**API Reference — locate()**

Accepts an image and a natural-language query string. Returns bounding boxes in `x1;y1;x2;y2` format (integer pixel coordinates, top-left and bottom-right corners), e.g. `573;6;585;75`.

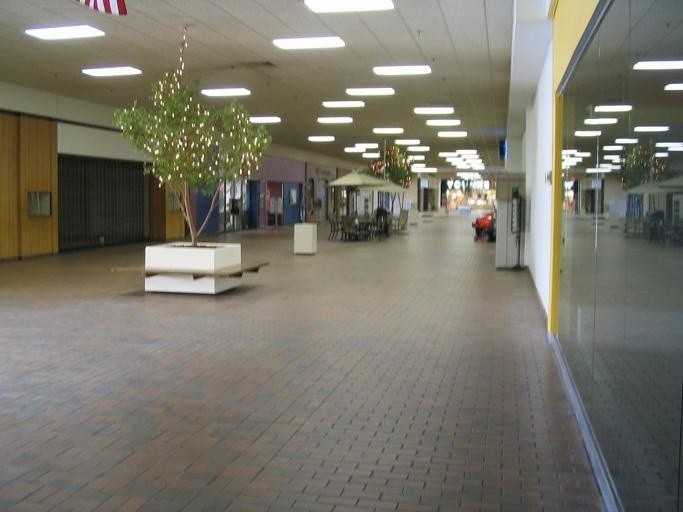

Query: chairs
327;210;410;241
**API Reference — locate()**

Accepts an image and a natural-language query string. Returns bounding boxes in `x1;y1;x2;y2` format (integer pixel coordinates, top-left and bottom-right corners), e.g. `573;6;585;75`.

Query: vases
146;239;242;296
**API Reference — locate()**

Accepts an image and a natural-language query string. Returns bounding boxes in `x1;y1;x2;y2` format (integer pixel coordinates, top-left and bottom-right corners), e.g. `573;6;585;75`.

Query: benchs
218;261;268;279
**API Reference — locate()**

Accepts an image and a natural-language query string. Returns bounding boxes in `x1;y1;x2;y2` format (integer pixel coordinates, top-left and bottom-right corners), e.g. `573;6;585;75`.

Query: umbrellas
658;175;683;189
328;171;383;217
354;181;409;209
626;180;683;223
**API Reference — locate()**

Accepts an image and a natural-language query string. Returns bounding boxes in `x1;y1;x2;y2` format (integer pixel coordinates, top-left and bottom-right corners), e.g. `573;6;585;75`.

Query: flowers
113;24;272;245
